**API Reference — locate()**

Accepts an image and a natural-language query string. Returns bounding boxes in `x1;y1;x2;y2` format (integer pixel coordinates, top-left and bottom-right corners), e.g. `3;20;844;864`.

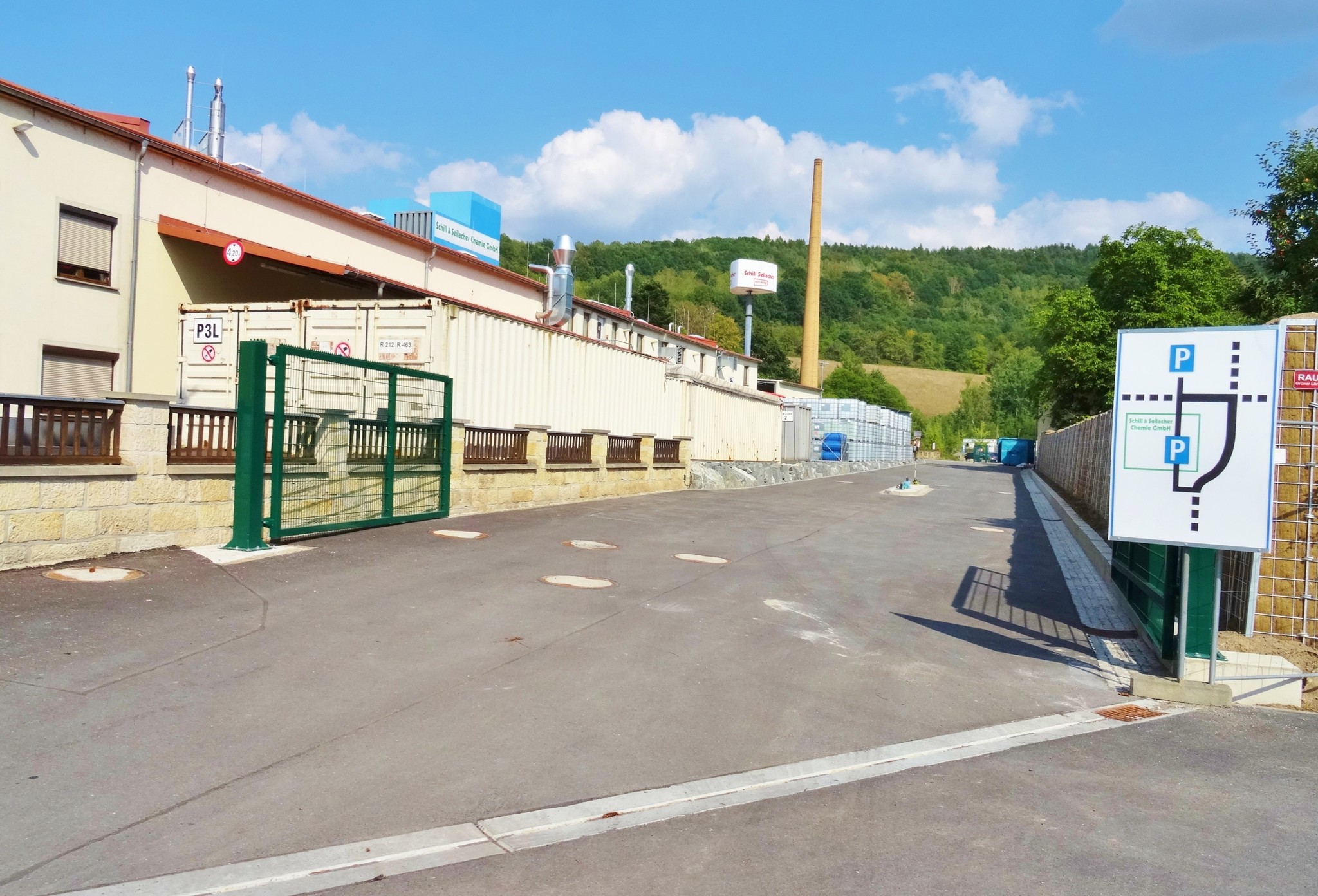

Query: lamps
12;120;34;133
320;277;362;290
258;258;309;278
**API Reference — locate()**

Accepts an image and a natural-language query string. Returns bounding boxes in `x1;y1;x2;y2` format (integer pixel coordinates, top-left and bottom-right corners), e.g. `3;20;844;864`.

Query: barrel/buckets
821;433;849;461
